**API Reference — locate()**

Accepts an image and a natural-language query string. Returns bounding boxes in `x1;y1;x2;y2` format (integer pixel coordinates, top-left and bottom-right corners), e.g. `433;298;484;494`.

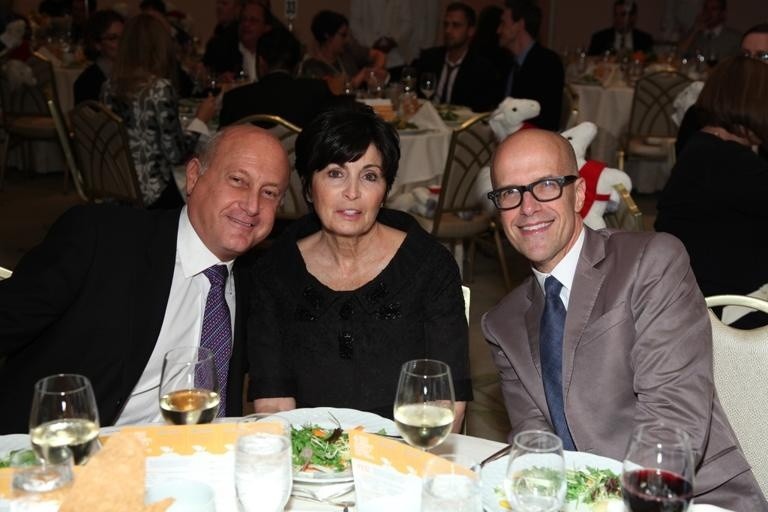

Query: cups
572;45;721;87
234;410;295;512
504;430;569;512
623;421;695;512
205;73;223;97
32;373;102;468
10;446;76;512
158;353;222;424
391;361;456;456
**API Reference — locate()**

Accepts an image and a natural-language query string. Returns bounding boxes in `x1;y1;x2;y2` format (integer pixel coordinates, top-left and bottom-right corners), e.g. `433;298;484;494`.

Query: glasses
744;51;768;61
488;175;577;211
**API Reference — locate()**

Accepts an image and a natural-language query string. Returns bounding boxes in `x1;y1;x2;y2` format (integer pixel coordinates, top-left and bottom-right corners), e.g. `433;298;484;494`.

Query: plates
254;404;408;485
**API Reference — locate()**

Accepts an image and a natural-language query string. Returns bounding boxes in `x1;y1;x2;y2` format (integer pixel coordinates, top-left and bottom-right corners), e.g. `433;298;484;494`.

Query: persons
648;53;768;330
0;124;290;436
479;127;767;510
382;1;564;130
585;1;766;59
246;93;476;435
1;2;386;210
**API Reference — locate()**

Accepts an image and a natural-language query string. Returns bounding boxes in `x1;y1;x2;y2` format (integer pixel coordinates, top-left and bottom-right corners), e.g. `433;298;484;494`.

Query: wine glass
421;453;486;512
343;68;464;135
477;450;647;512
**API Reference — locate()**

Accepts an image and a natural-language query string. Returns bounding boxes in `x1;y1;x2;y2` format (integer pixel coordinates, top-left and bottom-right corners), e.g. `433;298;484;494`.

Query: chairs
705;293;768;503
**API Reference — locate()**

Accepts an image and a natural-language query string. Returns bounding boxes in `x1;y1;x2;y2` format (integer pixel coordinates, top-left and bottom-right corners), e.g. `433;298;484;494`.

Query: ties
195;264;234;419
440;64;457;103
541;276;577;451
